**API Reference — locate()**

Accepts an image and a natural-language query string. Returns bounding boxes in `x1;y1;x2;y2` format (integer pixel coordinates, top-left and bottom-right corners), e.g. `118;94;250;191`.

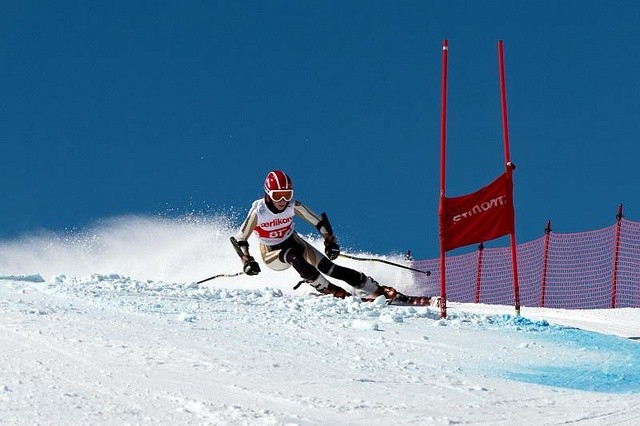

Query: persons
230;170;396;299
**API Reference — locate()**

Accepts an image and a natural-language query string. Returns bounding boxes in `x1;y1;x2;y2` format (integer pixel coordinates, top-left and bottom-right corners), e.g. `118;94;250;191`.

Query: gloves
325;243;340;260
243;259;261;275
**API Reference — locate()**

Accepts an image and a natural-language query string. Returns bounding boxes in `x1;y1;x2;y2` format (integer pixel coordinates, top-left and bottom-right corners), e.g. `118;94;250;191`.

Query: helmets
265;171;293;190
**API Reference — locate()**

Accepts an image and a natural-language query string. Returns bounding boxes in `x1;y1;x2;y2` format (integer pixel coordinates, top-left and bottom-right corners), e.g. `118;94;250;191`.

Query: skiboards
312;293;442;308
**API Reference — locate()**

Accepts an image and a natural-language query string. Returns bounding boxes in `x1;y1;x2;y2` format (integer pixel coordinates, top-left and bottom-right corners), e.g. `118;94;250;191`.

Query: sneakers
353;273;397;300
294;273;347;298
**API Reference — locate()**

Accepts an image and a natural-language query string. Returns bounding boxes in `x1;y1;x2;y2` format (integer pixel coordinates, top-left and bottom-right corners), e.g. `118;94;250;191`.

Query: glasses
265;190;294;202
